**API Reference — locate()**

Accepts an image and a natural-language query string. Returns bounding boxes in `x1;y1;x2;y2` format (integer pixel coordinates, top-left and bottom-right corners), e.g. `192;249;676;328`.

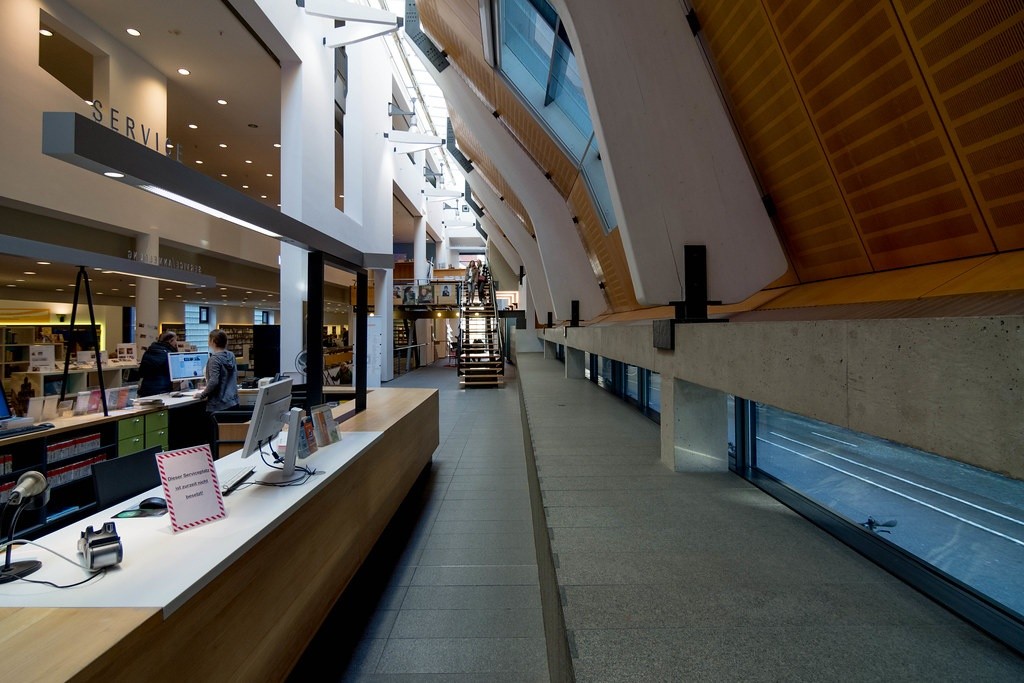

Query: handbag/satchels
478;275;486;281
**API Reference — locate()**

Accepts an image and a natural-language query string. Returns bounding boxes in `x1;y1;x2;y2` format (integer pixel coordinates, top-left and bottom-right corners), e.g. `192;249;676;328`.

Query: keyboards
218;465;256;494
0;425;49;439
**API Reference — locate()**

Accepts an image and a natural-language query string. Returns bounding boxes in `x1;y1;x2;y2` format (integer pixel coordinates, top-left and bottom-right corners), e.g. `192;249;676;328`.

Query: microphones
8;470;46;505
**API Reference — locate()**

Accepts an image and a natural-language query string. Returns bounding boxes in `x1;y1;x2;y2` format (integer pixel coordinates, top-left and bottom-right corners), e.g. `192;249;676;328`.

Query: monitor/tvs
0;379;12;420
167;351;209;391
240;377;308;486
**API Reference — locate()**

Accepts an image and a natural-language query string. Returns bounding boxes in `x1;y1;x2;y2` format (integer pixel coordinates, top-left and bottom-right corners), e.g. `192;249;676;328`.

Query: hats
159;331;176;342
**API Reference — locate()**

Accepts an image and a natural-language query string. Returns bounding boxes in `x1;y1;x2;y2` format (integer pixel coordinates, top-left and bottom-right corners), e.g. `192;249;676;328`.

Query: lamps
0;471;52;584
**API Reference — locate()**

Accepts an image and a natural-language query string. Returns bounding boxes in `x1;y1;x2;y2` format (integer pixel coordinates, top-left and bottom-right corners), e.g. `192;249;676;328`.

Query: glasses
409;291;413;294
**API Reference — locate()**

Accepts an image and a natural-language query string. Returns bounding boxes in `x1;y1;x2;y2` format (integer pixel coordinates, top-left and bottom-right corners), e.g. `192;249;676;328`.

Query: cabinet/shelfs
0;325;64;392
393;319;411;344
219;325;253;356
11;365;141;397
0;409;168;504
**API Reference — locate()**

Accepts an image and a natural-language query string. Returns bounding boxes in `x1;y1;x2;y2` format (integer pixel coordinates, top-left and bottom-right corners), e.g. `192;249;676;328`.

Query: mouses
40;422;55;428
139;497;167;508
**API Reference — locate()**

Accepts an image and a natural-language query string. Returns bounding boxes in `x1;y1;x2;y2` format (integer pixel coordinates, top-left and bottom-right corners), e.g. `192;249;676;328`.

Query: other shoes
471;304;475;307
466;300;470;305
479;303;484;307
484;298;487;304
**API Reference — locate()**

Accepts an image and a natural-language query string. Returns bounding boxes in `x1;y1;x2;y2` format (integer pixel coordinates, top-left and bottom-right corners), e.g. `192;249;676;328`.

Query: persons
193;329;241;420
135;330;179;398
341;328;348;347
476;260;487;306
330;361;352;385
402;287;414;304
442;286;449;296
465;260;478;306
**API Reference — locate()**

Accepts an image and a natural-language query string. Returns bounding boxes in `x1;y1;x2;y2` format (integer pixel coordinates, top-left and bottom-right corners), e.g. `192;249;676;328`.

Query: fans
295;349;326;376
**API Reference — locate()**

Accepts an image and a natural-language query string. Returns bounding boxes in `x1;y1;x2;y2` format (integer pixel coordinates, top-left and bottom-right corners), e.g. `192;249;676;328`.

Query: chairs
91;444;164;513
